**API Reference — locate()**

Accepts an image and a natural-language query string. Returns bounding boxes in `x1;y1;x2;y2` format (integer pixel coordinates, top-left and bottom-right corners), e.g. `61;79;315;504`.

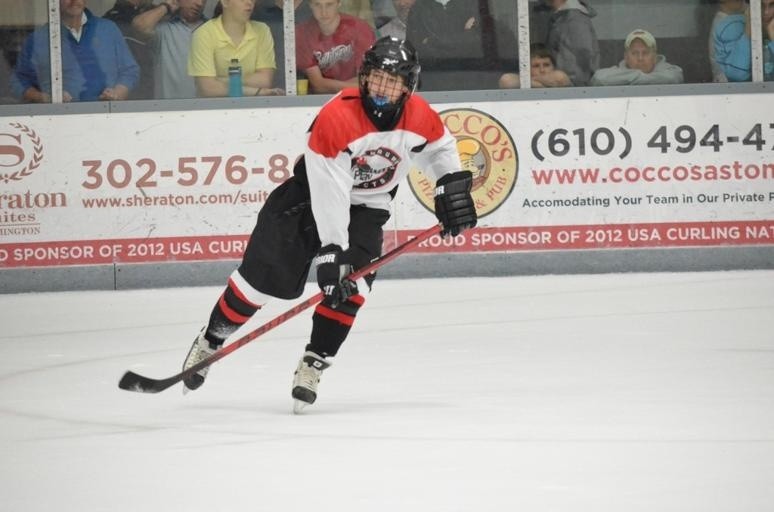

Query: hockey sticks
119;224;444;394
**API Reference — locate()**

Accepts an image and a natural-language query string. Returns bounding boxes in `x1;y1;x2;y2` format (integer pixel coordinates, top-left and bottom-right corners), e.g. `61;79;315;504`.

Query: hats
624;29;656;48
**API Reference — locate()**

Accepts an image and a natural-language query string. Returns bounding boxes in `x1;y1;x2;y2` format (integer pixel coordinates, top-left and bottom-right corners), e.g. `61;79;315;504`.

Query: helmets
359;35;419;122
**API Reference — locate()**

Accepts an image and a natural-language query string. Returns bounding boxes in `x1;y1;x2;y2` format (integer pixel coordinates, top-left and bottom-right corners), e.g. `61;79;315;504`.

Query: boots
182;328;224;390
291;344;330;404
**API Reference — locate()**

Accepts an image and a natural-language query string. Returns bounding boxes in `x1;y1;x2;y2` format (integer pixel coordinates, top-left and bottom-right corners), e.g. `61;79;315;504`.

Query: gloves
434;170;476;237
315;244;358;304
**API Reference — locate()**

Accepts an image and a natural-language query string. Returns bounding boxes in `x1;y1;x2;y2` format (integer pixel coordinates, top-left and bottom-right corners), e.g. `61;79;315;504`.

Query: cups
297;79;308;96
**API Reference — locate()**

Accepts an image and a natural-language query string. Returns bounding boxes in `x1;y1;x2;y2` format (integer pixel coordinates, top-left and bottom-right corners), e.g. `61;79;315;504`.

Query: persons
590;30;684;89
498;42;572;91
102;1;503;99
709;2;773;82
546;1;601;88
183;36;480;406
7;0;141;103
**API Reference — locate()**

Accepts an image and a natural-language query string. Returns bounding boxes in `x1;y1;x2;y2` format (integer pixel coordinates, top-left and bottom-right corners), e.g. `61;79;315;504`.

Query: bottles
228;59;243;97
